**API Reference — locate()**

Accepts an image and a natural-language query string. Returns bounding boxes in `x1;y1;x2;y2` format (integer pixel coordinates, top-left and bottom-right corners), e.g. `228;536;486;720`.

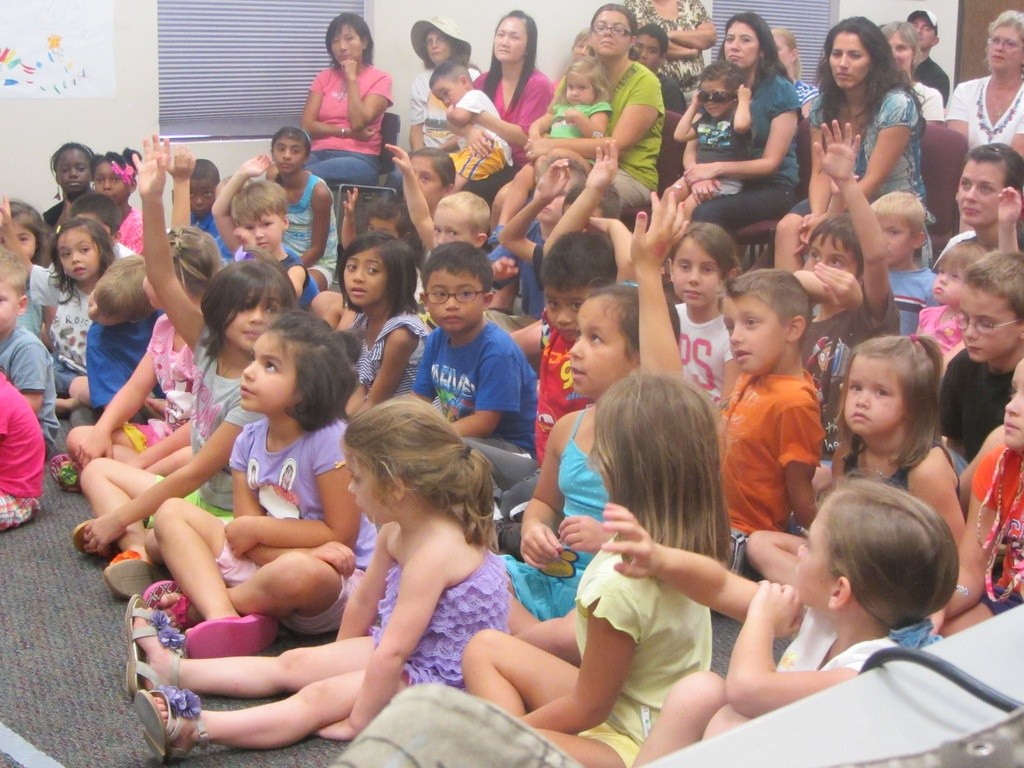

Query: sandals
134;686;209;763
125;593;185;697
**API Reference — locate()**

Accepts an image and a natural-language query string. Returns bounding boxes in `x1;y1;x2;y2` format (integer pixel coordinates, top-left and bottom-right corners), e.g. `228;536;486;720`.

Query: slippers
182;614;279;659
144;581;205;629
50;454;82;492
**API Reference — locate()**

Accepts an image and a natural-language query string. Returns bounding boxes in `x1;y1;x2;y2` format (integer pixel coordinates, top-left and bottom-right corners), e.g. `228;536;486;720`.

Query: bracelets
340;128;345;138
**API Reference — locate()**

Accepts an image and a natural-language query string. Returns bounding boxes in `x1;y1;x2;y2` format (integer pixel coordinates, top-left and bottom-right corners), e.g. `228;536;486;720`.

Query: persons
461;378;731;768
122;393;508;766
0;0;1024;766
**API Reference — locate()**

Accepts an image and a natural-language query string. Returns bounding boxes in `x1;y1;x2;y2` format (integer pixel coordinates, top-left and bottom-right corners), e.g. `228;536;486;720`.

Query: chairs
620;109;969;268
331;113;400;231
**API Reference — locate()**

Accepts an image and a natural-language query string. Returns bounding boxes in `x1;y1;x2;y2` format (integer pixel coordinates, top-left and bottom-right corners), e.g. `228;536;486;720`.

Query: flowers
112;162;134;186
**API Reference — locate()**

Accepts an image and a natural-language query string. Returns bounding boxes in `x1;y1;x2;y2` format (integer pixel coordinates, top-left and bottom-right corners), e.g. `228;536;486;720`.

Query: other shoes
104;551;167;600
70;408;96;426
72;519;121;558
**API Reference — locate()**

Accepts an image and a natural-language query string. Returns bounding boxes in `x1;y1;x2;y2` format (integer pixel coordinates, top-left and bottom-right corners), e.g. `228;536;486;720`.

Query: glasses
987;36;1022;49
592;25;633;36
698;91;736;102
954;312;1021;334
424;290;484;303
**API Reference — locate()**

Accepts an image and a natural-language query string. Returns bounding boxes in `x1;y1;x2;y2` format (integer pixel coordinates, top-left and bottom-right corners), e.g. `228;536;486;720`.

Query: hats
908;11;937;36
411;21;471;65
